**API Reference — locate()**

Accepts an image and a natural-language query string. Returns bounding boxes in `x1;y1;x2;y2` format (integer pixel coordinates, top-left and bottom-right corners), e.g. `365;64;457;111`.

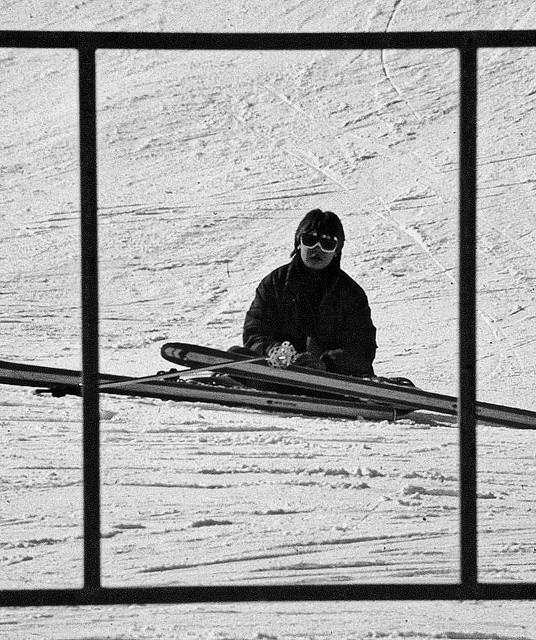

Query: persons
224;210;378;382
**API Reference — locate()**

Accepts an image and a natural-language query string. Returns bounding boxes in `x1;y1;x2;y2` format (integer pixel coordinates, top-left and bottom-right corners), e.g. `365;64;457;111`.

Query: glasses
298;231;339;254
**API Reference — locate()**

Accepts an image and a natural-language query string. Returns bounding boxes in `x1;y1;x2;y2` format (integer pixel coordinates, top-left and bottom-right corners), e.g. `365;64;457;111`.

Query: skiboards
0;342;534;431
